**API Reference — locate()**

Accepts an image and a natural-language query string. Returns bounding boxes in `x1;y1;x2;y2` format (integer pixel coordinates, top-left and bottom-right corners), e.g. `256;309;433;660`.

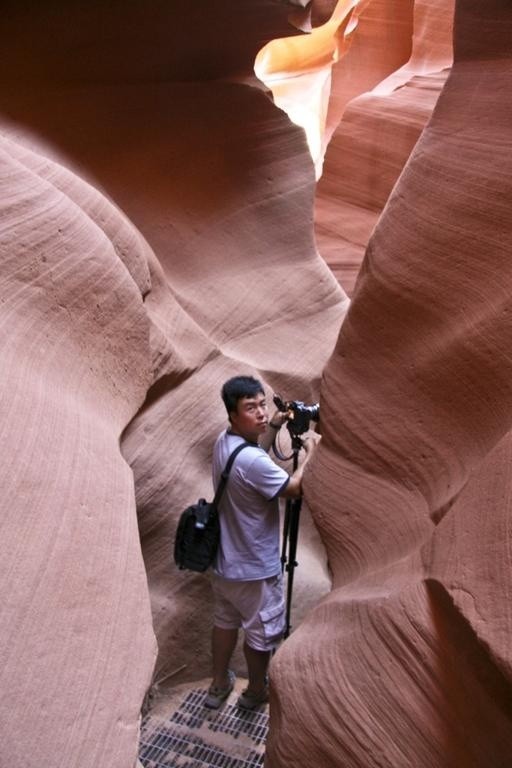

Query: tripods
280;438;304;640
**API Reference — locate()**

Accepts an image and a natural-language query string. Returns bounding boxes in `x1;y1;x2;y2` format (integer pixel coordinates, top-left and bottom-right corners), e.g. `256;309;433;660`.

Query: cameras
284;399;320;436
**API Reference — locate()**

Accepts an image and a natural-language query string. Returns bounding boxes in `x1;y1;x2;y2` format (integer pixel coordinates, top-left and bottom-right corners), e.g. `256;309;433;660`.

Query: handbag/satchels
173;441;256;574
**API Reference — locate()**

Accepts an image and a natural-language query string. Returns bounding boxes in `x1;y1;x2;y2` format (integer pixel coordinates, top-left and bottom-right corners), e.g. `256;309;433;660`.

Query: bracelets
270;421;283;432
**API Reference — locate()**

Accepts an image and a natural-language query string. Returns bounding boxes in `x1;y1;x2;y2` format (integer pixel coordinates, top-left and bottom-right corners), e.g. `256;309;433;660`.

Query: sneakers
235;675;270;711
203;666;235;710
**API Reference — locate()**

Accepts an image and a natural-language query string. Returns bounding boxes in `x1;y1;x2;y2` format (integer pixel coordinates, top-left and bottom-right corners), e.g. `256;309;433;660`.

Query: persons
199;373;313;713
